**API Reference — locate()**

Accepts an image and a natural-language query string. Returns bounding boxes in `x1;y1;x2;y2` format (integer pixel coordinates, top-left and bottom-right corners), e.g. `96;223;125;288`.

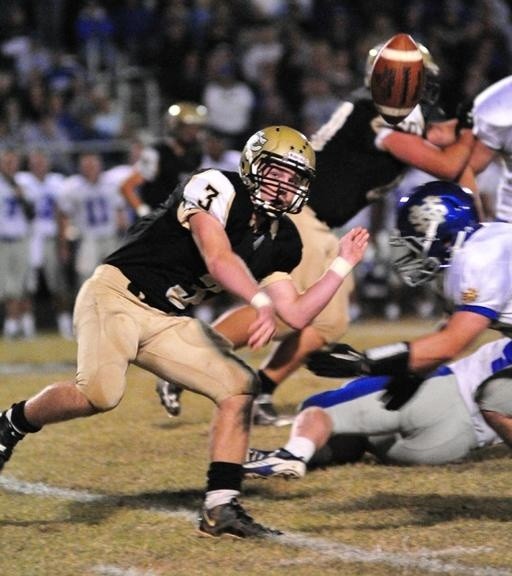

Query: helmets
163;101;209;144
238;125;317;217
387;180;479;287
363;39;441;110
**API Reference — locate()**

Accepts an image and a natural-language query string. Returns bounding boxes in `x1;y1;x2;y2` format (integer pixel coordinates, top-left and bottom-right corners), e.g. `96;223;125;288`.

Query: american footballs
370;34;425;125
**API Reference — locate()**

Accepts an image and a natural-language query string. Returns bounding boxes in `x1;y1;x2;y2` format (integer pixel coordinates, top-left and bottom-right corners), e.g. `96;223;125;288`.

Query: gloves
380;373;423;412
301;341;410;378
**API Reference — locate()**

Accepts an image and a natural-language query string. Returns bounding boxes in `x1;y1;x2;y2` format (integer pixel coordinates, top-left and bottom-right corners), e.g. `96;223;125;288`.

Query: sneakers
250;394;295;428
156;379;183;415
197;497;282;541
242;447;308;479
0;408;24;470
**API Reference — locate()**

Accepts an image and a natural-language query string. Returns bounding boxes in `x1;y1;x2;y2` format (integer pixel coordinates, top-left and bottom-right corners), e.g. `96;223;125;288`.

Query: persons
155;36;512;477
1;125;369;539
0;0;512;338
